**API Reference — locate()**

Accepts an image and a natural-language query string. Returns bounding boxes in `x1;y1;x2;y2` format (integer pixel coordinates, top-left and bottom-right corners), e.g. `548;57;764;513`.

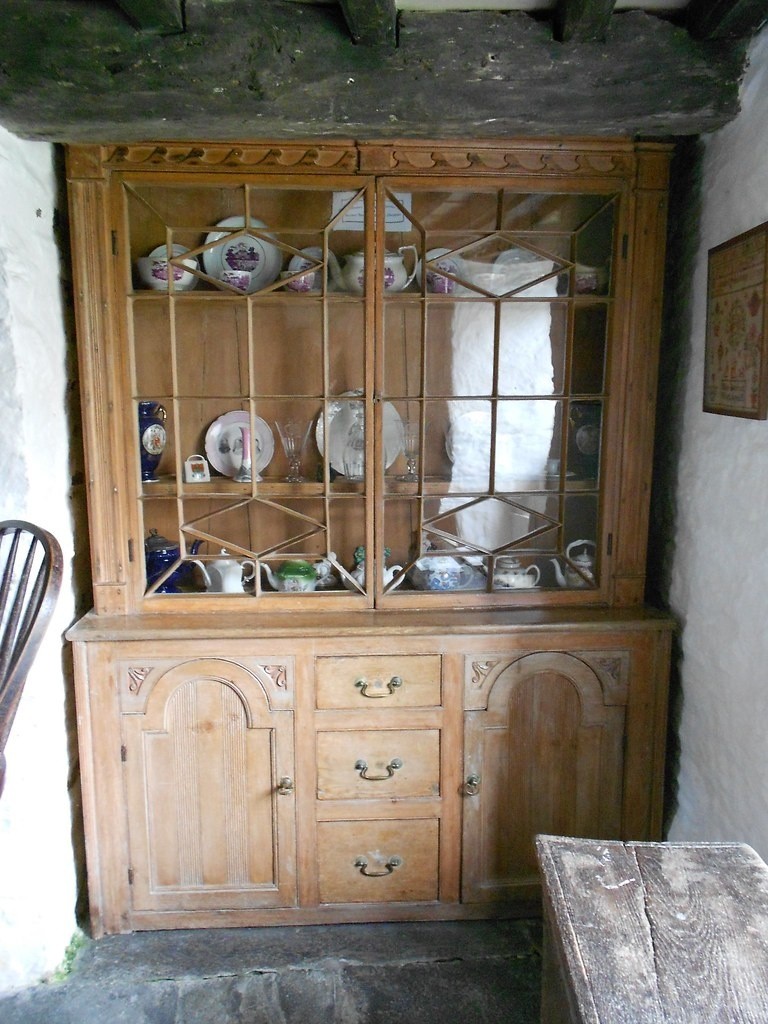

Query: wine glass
395;420;427;482
274;420;313;483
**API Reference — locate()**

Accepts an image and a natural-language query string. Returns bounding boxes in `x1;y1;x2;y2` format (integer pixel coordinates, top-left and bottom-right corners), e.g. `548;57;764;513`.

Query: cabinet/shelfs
60;137;679;922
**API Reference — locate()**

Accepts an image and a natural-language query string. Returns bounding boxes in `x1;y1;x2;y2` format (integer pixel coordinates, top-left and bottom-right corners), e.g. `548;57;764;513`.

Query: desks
533;834;768;1024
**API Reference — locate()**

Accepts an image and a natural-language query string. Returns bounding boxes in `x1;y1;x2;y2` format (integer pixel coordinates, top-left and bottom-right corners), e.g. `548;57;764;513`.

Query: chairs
0;519;62;798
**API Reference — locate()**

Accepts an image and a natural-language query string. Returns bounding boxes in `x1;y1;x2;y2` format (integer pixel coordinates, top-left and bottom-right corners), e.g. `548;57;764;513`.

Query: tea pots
318;242;417;292
144;528;599;593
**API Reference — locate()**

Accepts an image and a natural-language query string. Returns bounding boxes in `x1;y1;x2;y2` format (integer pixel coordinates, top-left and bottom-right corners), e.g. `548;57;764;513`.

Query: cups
547;459;560;475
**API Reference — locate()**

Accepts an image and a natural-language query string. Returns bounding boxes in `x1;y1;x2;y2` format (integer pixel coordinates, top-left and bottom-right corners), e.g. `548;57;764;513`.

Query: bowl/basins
431;273;456;293
138;258;197;290
218;271;251;292
280;271;315;292
575;267;603;295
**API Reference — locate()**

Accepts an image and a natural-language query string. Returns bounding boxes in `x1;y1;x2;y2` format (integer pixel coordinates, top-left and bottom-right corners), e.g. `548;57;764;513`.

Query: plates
446;412;491;477
492;248;538;296
149;244;200;290
416;248;468;293
289;247;338;292
544;471;575;478
316;391;403;475
203;216;282;294
205;410;274;476
